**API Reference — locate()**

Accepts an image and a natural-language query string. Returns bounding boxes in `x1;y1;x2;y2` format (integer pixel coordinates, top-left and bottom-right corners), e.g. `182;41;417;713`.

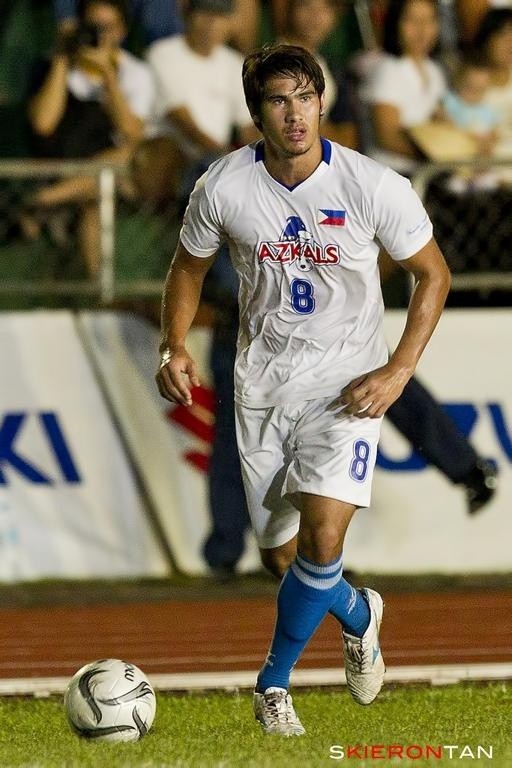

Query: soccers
63;657;157;745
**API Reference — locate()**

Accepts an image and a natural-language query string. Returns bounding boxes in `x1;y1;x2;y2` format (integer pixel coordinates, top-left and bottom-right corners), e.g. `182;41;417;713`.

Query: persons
2;0;512;281
153;36;451;739
175;117;502;580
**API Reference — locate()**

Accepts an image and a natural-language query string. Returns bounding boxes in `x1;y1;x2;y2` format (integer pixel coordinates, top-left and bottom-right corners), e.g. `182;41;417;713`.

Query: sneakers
250;684;307;738
337;584;388;707
